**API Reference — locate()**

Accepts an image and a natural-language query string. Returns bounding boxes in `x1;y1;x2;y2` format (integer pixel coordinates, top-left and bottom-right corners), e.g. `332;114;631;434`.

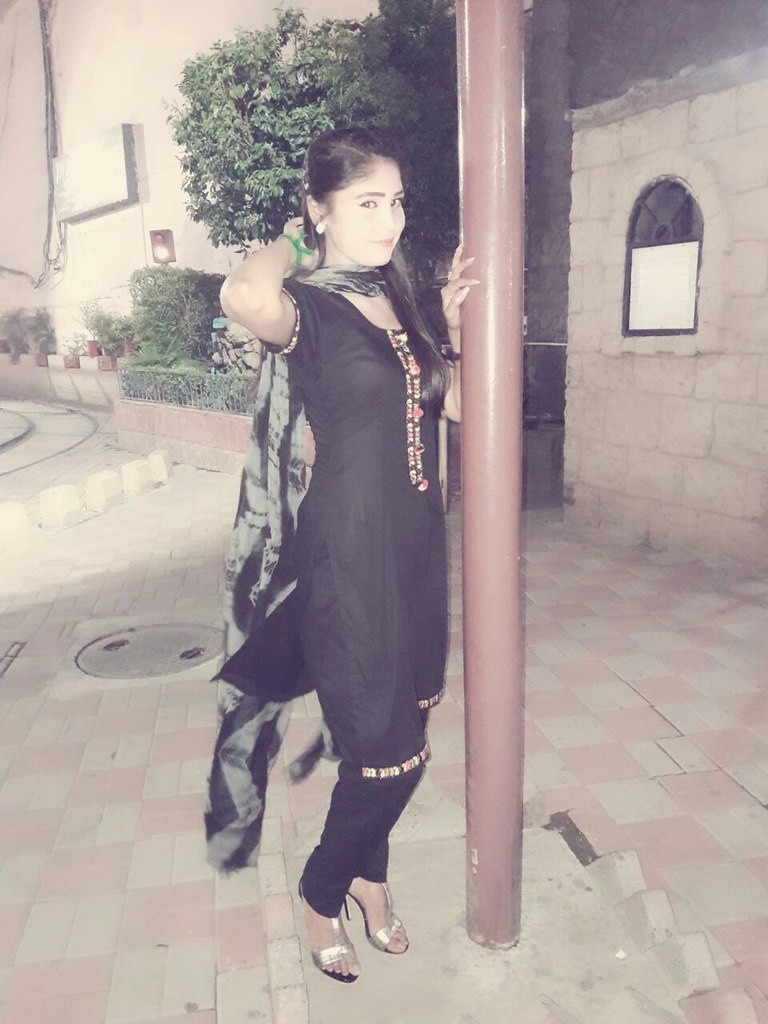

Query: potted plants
80;297;141;373
0;309;24;367
24;305;57;367
60;336;81;370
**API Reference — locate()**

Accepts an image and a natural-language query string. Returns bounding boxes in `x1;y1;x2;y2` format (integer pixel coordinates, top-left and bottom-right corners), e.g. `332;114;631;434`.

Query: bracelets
278;232;313;266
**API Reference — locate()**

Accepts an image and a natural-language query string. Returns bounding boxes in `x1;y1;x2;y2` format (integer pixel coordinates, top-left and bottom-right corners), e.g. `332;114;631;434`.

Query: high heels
299;876;358;984
344;883;409;954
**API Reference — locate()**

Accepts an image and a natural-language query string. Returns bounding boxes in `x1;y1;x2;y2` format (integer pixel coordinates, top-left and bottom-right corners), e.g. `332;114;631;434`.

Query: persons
204;126;480;986
